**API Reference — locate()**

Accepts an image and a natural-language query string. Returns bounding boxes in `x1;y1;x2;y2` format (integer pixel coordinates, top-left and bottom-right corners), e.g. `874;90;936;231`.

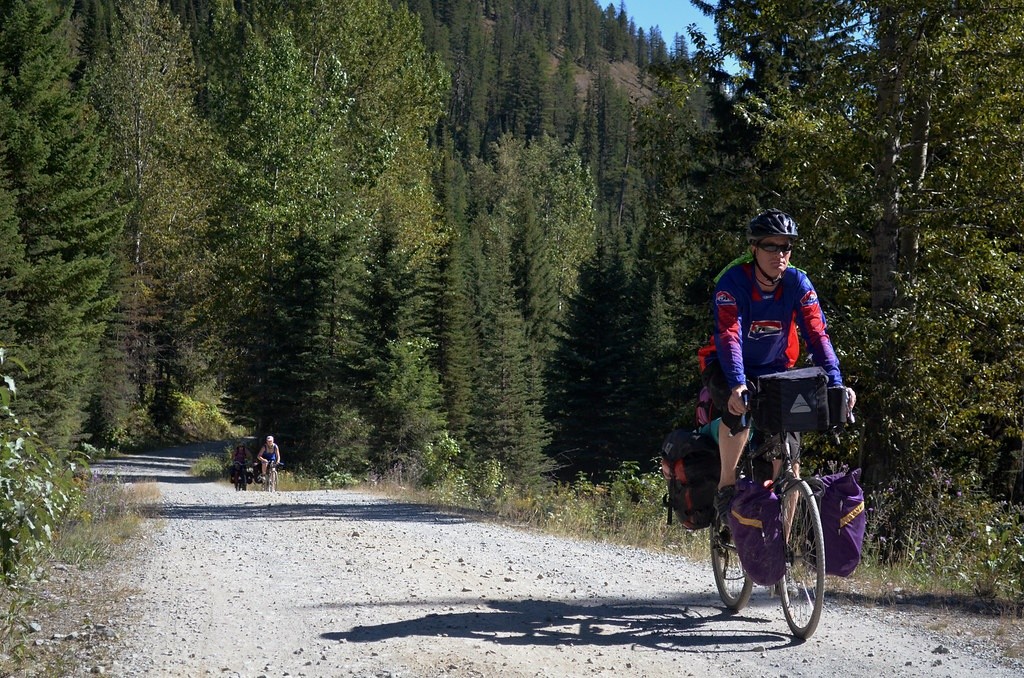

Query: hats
267;436;274;442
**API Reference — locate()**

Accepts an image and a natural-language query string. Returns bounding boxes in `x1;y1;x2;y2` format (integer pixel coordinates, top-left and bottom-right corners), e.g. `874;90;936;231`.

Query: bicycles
260;458;278;493
232;460;250;491
709;374;856;641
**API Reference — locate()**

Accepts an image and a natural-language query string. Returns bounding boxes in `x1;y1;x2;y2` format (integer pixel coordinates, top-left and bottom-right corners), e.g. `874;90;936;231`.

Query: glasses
750;242;793;252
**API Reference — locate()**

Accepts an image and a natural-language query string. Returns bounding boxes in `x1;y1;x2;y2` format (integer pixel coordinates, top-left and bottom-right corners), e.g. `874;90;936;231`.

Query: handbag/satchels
726;477;788;586
661;430;722;530
757;366;829;434
804;468;865;577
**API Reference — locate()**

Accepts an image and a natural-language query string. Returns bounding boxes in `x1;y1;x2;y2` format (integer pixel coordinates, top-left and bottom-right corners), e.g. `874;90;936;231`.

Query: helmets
746;208;798;246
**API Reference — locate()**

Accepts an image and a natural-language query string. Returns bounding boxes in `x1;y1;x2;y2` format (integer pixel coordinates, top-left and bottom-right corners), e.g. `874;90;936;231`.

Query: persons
232;441;253;482
702;206;859;573
257;435;281;485
